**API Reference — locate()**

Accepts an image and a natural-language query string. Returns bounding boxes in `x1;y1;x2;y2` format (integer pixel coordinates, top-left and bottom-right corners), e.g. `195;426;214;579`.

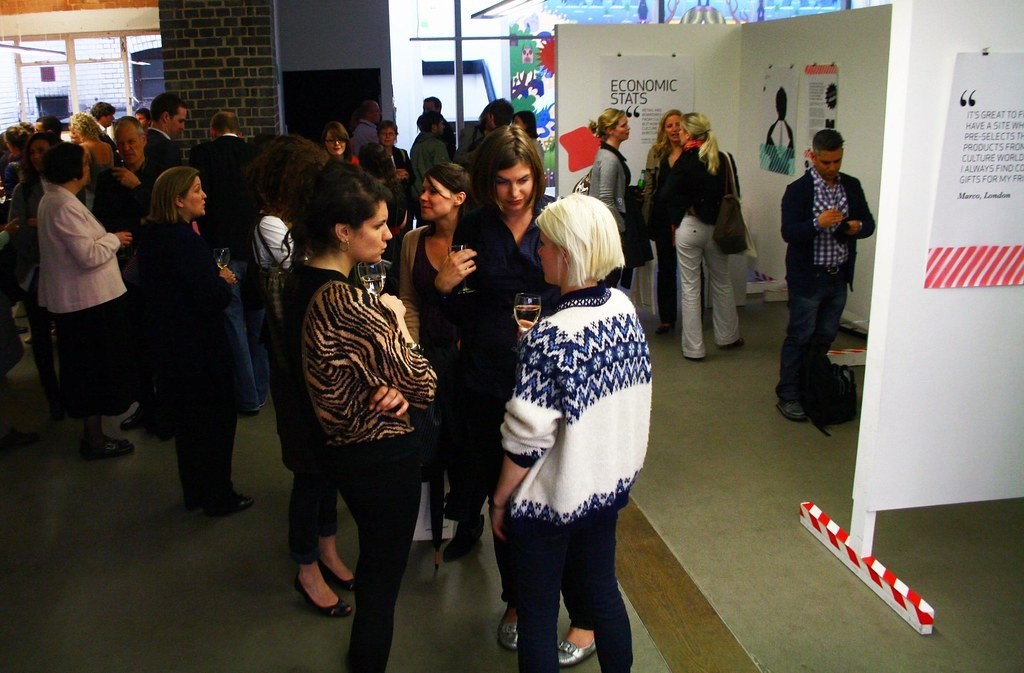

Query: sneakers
777;399;806;419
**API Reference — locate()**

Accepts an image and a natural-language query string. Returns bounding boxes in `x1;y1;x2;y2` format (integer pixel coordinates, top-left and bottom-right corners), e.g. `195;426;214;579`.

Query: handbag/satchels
712;150;749;254
240;260;275;297
801;354;856;436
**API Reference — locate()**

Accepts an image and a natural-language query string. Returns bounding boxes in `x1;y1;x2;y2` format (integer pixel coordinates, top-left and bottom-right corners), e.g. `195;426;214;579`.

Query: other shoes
0;427;40;447
204;494;253;516
497;607;519;650
655;323;672;334
442;514;485;561
15;327;27;334
557;637;596;666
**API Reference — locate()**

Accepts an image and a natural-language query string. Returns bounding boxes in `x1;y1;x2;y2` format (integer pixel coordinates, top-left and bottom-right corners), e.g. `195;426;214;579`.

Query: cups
357;259;386;296
213;248;230;269
111;159;124;167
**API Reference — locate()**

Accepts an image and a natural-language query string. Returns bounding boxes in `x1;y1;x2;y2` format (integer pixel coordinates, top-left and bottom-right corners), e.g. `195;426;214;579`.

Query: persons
492;193;652;673
0;92;187;460
643;109;705;334
587;109;632;288
270;158;437;673
135;166;259;517
431;125;596;666
776;129;876;420
657;113;745;360
189;110;331;280
399;162;485;561
324;97;543;236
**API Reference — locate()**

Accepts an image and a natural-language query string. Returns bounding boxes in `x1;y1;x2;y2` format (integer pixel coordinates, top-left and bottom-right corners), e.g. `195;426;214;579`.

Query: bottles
638;170;645;188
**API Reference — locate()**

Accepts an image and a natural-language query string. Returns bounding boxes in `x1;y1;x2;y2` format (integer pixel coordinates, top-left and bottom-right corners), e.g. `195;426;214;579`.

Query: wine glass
511;293;541;352
448;245;475;294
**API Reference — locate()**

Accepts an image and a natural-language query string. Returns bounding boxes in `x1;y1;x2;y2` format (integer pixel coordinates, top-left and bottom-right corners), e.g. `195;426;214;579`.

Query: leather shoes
121;407;147;428
79;436;134;459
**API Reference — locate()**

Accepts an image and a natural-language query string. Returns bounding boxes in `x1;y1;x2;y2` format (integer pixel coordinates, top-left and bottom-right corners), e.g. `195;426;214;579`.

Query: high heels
316;553;355;590
294;577;351;616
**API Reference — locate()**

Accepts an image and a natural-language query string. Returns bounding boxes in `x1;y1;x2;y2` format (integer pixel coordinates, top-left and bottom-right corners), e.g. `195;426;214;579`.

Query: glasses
325;138;344;143
